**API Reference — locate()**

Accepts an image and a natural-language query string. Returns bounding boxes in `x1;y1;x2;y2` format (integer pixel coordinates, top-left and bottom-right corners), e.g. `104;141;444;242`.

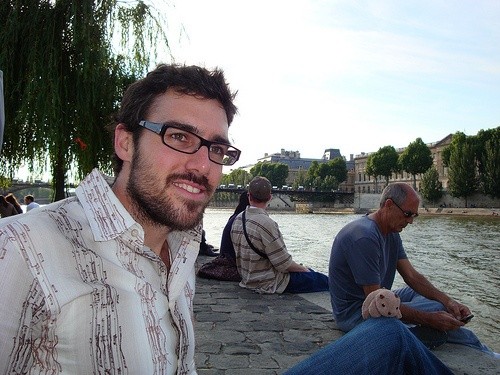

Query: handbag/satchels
198;253;241;281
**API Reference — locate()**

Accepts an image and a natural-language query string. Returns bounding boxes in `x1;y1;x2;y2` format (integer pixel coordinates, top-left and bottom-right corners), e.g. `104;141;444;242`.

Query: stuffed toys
362;289;402;320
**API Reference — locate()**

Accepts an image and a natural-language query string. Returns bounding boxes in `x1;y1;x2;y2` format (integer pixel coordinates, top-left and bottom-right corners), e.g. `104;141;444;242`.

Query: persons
231;176;328;294
328;182;500;358
5;193;23;214
220;192;250;259
0;195;17;218
0;65;456;375
24;195;39;211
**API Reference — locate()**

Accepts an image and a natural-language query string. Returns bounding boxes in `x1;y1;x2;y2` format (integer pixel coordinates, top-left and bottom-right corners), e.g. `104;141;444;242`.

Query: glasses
139;120;241;166
385;197;419;218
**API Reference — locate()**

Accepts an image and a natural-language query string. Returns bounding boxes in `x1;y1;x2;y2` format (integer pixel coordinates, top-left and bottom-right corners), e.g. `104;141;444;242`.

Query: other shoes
408;325;447;349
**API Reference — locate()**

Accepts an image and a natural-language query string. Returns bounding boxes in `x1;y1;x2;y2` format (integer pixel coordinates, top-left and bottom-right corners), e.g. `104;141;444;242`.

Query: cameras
459;314;474;322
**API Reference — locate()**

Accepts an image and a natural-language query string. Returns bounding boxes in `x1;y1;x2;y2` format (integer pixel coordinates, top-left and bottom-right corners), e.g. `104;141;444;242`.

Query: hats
249;176;271;199
